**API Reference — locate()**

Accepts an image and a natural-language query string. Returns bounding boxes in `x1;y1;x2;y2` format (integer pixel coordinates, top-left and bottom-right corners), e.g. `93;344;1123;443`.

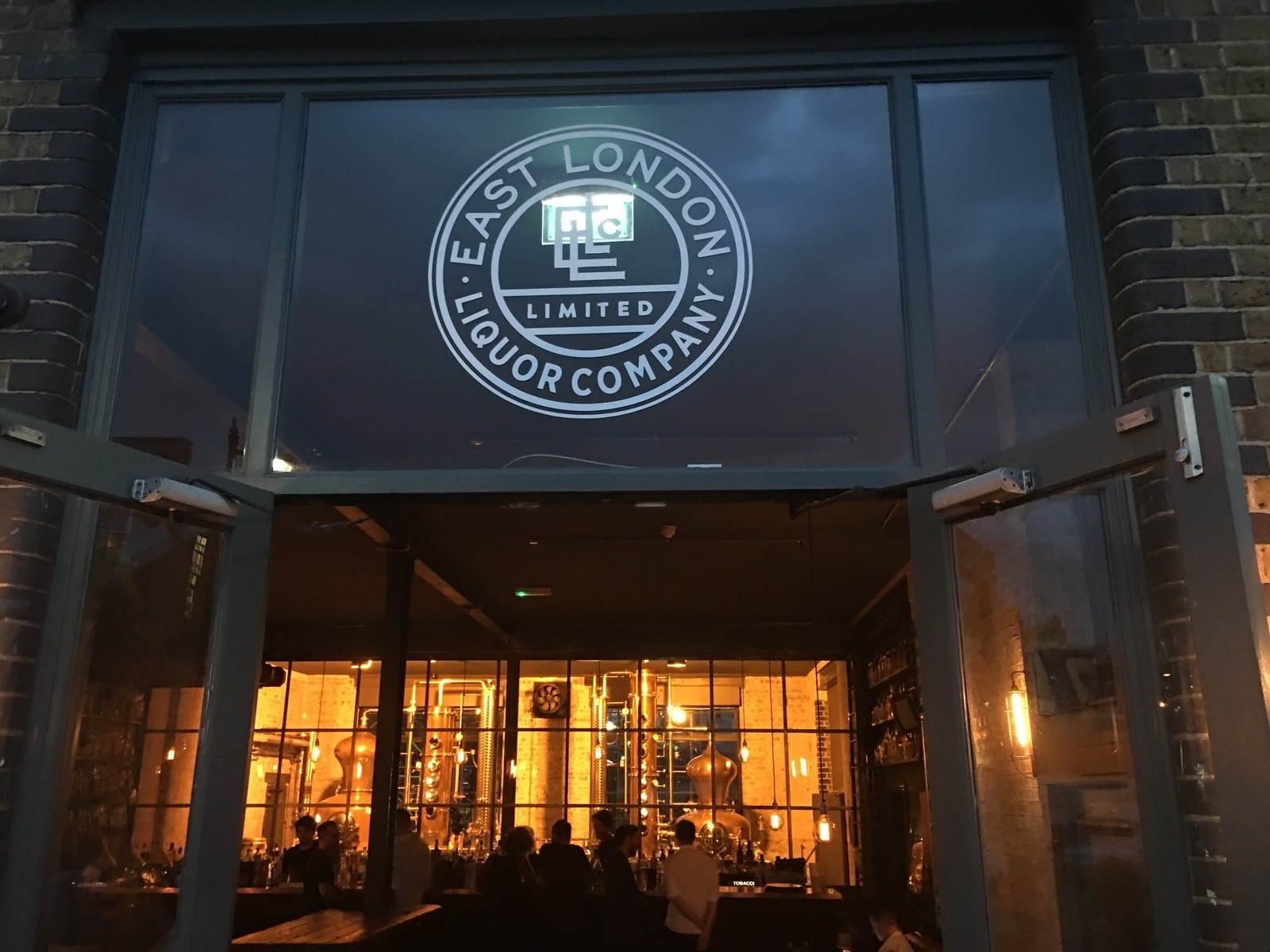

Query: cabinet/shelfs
867;663;922;768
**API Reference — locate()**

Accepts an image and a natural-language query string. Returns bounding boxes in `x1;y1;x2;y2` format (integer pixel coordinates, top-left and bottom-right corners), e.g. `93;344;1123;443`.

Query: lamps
1004;671;1038;780
738;661;751;764
812;661;837;844
767;660;784;830
454;660;468;765
310;662;322;762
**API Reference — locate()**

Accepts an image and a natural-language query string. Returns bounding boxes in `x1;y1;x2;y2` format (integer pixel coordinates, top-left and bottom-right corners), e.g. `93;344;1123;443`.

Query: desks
231;904;441;952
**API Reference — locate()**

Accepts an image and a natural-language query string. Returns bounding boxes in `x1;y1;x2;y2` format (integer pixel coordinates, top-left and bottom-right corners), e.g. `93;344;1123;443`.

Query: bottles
240;843;369;891
584;846;591;862
713;839;783;882
866;638;924;765
428;836;501;866
133;842;183;863
639;848;677;893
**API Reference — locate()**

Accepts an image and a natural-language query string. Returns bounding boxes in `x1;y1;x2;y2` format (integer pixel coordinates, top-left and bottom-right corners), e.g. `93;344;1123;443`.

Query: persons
392;808;434;906
475;827;550;952
660;819;720;952
838;899;915;952
268;816;319;886
539;819;589;952
589;809;652;952
303;821;340;914
